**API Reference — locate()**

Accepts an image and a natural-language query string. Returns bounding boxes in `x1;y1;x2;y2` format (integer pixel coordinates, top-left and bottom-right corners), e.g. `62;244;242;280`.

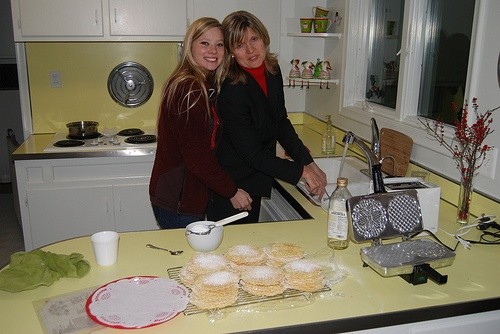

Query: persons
206;10;327;225
149;17;252;228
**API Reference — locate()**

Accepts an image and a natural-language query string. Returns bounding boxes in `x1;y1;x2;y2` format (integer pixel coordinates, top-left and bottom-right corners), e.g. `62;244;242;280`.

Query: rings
248;197;251;201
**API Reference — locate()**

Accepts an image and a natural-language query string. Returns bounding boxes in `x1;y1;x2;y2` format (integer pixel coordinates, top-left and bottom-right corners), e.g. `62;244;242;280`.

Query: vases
456;177;473;224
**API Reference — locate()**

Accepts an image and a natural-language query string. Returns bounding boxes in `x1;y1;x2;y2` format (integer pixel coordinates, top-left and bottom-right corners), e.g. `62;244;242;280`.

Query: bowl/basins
185;220;223;251
67;120;100;137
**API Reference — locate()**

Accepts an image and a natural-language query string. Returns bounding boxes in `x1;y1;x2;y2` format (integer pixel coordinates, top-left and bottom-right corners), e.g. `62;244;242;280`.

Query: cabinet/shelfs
11;0;349;86
15;160;305;254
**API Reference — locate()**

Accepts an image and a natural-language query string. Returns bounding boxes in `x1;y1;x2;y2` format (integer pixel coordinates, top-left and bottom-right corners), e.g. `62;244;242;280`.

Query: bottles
321;115;336;155
327;177;353;250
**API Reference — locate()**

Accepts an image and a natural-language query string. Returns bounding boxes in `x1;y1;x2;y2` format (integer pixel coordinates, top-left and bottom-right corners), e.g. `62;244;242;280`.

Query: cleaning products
320;114;336;155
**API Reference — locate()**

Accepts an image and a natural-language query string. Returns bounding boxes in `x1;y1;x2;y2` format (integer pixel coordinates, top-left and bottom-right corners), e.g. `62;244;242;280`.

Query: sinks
289;158;363;184
322;182;371;212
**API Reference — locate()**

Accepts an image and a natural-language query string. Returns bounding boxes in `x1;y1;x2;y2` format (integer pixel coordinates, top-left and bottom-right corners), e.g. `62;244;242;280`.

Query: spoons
147;244;183;255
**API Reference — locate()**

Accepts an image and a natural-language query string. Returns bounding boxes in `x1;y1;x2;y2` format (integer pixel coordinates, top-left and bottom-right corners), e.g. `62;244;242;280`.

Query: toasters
371;176;442;236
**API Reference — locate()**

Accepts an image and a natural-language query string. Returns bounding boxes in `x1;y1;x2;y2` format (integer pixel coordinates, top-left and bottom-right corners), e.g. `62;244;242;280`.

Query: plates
86;276;181;329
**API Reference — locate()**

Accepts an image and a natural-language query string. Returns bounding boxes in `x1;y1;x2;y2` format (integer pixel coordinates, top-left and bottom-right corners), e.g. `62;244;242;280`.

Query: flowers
417;97;500;220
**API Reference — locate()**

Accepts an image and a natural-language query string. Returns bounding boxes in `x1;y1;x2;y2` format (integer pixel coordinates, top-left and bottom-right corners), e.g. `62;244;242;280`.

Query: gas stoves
44;128;159;149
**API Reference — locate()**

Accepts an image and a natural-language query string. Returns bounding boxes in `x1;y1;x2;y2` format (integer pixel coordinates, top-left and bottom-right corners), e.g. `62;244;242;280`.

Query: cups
91;231;119;266
300;6;333;35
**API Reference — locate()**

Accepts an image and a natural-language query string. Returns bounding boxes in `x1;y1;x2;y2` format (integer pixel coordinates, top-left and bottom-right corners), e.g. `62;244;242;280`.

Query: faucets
342;117;381;175
